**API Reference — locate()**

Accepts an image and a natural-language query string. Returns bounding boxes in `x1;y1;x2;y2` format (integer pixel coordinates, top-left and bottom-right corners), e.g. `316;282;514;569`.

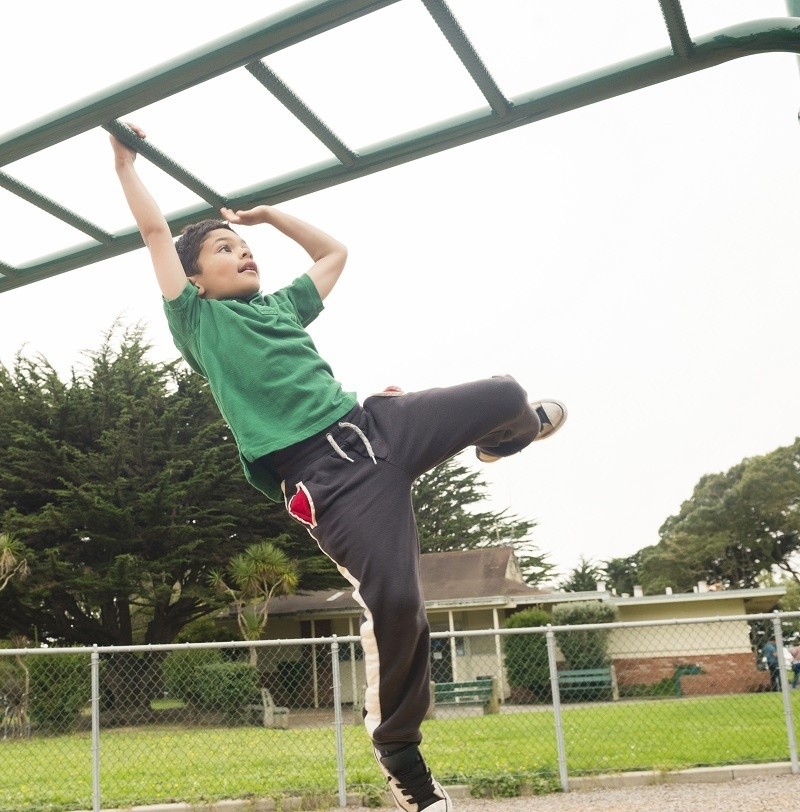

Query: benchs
557;665;620;701
424;677;499;718
244;688;290;729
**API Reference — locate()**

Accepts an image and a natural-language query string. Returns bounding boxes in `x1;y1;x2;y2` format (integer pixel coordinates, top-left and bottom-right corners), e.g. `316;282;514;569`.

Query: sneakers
475;402;566;463
373;742;452;812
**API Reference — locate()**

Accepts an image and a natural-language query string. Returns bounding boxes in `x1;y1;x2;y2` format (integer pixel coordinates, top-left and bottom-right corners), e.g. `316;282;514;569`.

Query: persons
764;636;782;692
108;122;567;812
779;640;794;672
790;638;800;689
